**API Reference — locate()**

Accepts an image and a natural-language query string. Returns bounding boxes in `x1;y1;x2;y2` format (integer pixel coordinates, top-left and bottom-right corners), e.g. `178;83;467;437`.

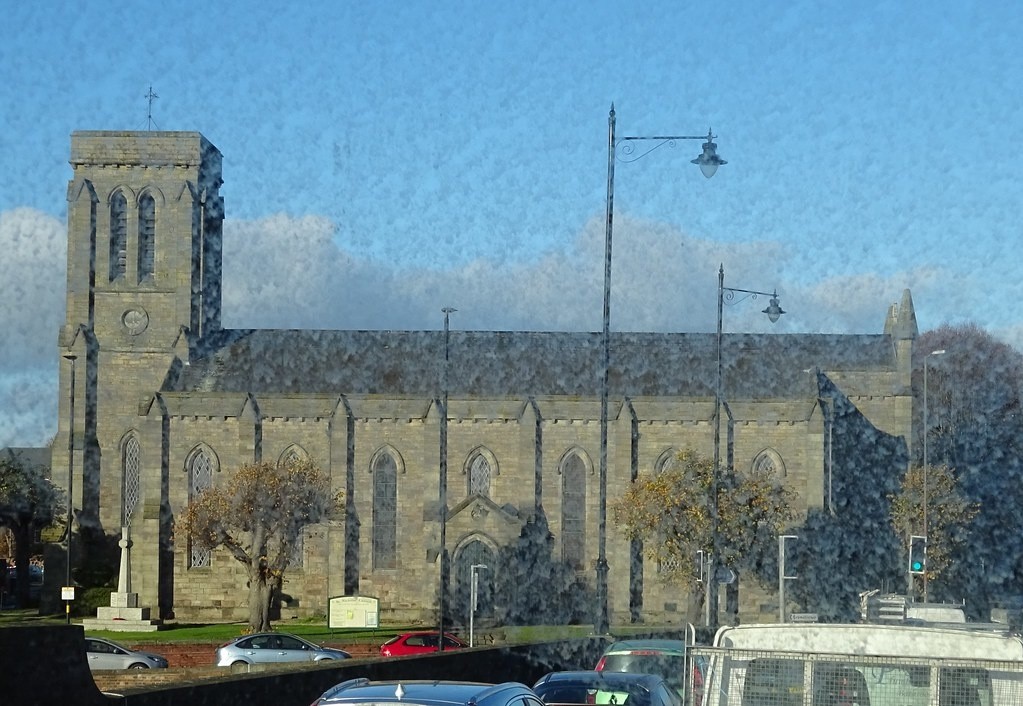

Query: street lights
920;342;947;607
63;346;76;585
440;306;453;650
595;101;728;636
713;262;783;623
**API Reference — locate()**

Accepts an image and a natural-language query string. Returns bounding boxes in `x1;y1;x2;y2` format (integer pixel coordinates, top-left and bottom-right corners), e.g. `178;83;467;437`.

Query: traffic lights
909;536;928;579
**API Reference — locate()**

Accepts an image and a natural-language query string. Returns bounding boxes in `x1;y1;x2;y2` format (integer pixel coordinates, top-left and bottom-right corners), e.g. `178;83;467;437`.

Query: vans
699;617;1016;706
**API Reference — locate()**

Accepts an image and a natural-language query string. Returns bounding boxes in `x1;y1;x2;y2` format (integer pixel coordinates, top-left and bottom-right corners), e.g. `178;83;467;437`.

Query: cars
209;635;350;670
534;671;686;705
594;639;709;697
87;636;167;675
378;630;469;655
313;679;541;706
